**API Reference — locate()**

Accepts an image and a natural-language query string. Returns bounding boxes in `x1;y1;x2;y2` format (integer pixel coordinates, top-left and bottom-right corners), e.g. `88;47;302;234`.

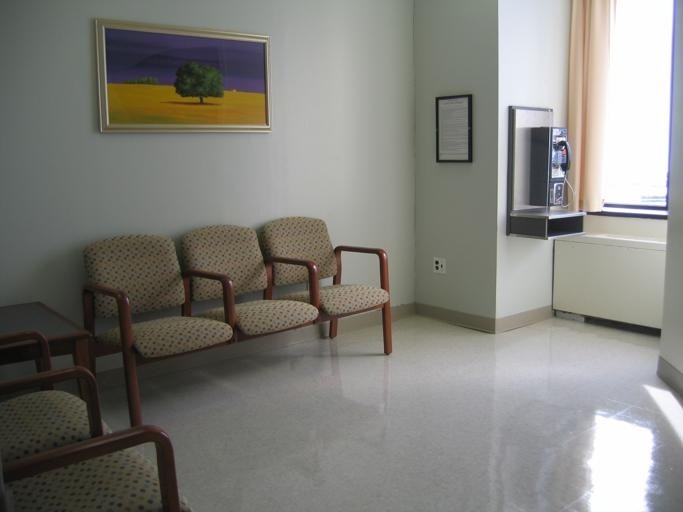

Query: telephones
550;127;571;182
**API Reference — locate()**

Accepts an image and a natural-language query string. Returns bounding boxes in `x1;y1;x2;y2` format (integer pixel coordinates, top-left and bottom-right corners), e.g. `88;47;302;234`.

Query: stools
0;329;190;512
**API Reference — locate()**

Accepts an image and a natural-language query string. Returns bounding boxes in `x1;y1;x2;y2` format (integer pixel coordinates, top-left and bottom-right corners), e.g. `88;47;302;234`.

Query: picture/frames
433;93;472;164
92;16;275;136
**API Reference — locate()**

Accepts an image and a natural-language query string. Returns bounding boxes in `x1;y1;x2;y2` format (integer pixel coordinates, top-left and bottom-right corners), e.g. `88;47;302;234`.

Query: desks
1;300;94;399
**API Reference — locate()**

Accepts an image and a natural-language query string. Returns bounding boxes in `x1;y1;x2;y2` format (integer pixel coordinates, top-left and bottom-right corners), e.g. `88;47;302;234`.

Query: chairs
82;215;393;397
